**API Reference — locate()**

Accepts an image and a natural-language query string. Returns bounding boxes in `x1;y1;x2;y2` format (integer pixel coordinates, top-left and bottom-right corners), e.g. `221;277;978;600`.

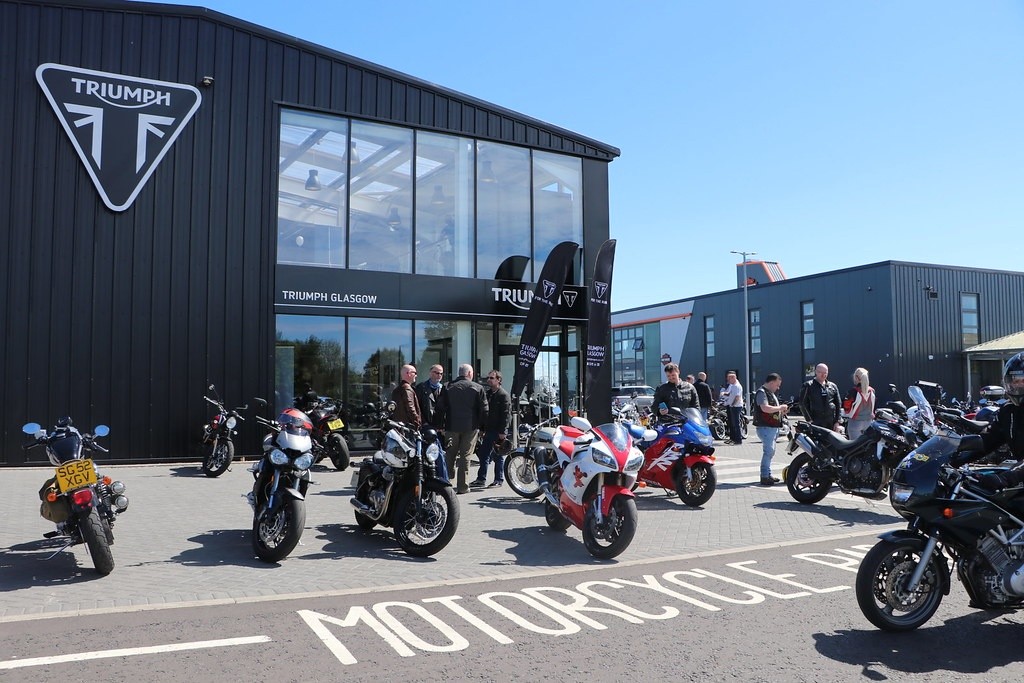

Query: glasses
486;377;498;379
407;372;417;375
435;372;443;375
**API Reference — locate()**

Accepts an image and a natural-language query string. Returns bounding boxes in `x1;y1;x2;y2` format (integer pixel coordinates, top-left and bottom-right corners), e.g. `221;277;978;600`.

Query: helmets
1003;351;1024;406
494;437;513;456
272;408;313;438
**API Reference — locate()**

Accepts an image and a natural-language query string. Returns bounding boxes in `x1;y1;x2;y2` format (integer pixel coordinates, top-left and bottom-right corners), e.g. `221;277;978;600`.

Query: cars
612;386;656;407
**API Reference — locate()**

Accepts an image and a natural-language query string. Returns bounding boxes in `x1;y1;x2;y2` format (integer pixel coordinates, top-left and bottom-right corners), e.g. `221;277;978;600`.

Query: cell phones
659;403;668;411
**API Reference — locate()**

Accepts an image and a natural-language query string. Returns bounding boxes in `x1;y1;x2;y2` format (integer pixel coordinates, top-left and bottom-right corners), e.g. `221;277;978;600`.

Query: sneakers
469;481;485;487
488;482;503;488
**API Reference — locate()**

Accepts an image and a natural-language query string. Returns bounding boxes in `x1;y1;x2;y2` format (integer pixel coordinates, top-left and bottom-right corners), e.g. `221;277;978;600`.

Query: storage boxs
980;386;1005;400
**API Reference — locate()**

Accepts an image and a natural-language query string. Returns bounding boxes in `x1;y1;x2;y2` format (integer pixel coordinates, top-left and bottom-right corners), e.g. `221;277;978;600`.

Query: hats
728;371;735;376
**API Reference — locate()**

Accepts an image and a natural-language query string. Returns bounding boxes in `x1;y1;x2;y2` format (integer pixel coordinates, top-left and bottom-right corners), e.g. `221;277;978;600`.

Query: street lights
731;250;759;416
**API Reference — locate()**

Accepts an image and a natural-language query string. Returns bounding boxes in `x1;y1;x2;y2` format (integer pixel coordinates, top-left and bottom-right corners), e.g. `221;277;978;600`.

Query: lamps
387;207;401;225
342;141;360;165
305;148;321;191
478;160;494;181
431;186;445;205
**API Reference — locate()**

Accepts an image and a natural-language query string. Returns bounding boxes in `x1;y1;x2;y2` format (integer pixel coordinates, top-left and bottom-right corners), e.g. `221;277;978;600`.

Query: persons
650;362;701;496
413;364;447;429
431;363;490;494
719;384;726;399
686;374;696;384
888;383;900;401
360;382;397;441
469;369;512;489
842;367;876;441
948;350;1024;608
693;371;712;422
799;363;842;435
720;369;743;446
752;372;789;487
391;364;422;432
710;387;717;401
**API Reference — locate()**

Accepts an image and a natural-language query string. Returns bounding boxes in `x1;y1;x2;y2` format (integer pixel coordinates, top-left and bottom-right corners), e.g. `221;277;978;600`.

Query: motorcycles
530;416;659;559
504;406;562;498
247;397;340;563
855;430;1024;632
23;416;129;575
202;383;249;478
612;391;795;442
349;399;460;557
293;391;349;471
630;407;717;507
782;381;1011;504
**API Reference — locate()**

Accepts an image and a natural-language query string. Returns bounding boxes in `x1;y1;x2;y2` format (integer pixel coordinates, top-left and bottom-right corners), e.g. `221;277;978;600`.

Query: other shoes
724;439;742;445
457;488;470;493
761;473;780;485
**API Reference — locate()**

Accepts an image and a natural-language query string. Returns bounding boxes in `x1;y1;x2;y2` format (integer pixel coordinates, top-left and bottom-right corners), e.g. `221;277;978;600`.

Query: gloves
979;473;1008;490
